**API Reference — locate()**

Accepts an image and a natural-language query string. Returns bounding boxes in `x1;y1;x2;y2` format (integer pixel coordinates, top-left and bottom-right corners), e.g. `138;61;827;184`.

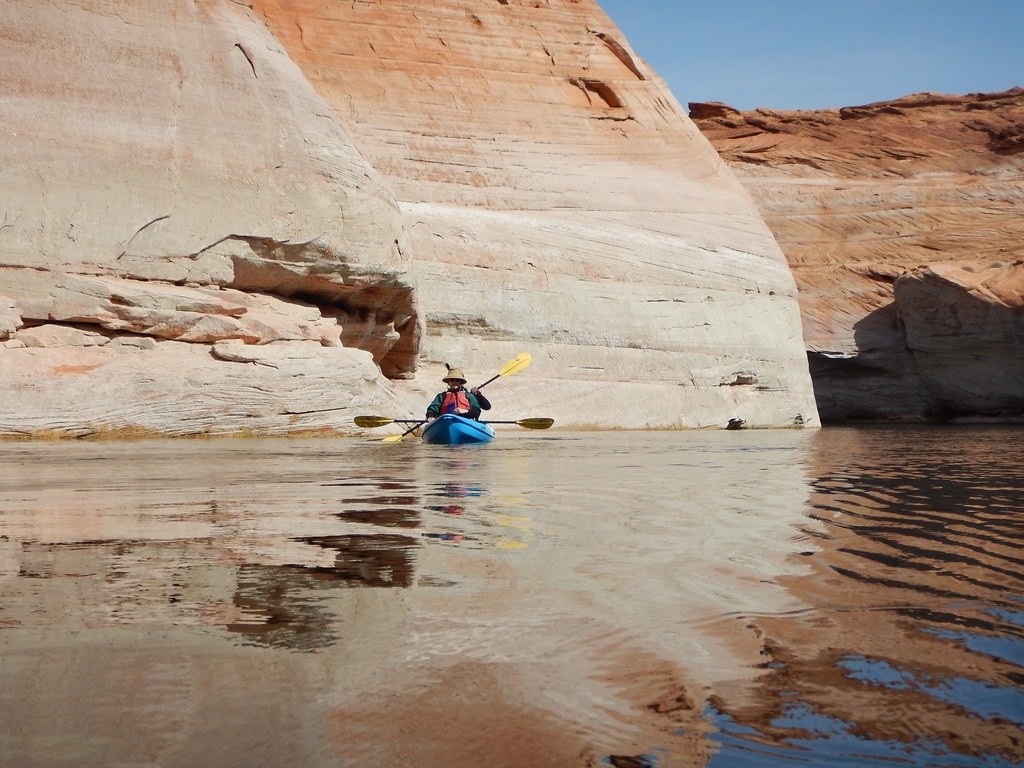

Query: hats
442;368;468;384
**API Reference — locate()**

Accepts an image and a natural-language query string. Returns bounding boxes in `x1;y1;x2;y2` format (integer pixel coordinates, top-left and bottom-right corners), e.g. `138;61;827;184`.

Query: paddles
353;416;554;429
382;352;531;441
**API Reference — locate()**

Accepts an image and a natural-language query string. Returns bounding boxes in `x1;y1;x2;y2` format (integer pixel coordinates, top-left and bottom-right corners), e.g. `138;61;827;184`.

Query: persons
425;368;492;422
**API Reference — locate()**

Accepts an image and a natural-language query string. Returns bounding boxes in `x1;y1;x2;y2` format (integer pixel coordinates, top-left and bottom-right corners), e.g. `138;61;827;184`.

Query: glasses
450;379;463;383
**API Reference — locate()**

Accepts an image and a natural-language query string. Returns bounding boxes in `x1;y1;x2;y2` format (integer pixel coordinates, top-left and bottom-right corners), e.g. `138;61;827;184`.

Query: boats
421;412;496;444
